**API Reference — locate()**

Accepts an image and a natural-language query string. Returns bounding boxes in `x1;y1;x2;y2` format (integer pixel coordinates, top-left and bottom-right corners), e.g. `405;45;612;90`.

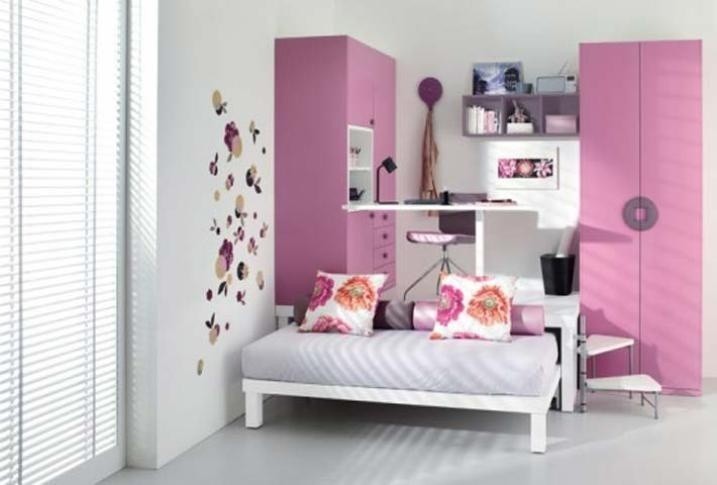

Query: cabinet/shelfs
461;92;580;137
579;312;663;419
275;36;395;307
579;39;702;396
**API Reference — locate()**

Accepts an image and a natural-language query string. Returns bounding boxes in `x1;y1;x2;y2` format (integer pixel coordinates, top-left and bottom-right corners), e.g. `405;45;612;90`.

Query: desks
347;202;540;277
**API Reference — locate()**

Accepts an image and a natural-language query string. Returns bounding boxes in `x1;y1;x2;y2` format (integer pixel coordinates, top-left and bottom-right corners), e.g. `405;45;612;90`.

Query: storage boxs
544;115;577;133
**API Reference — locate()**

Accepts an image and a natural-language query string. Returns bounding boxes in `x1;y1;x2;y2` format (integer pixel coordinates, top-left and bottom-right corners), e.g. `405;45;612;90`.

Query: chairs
403;192;488;301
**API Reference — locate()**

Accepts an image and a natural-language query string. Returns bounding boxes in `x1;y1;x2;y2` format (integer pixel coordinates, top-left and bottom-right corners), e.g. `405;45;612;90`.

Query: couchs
240;320;561;453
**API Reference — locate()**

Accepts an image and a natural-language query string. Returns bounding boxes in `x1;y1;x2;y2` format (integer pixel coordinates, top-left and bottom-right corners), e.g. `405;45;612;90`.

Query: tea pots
349;188;363;200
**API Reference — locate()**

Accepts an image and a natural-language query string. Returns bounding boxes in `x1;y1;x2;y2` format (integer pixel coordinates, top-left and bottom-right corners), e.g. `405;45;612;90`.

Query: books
465;105;499;133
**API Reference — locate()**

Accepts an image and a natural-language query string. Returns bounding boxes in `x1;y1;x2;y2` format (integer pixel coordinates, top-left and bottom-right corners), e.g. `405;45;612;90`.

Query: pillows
294;293;414;330
429;271;519;342
297;270;389;336
412;301;544;334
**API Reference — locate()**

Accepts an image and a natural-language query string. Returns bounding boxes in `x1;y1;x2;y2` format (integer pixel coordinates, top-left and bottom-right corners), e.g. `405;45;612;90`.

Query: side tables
487;276;580;412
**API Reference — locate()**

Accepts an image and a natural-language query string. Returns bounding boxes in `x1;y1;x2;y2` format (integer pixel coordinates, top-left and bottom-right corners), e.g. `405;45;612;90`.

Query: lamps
373;156;399;204
